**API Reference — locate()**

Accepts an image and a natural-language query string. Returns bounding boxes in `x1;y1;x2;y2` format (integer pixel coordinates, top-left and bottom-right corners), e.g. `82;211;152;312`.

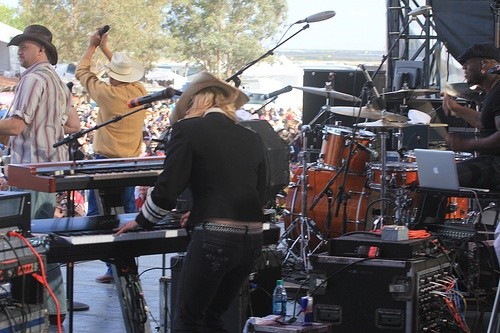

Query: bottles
272;280;287;322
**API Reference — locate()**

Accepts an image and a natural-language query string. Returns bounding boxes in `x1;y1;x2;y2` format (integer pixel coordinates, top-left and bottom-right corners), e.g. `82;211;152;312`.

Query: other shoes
95;273;115;284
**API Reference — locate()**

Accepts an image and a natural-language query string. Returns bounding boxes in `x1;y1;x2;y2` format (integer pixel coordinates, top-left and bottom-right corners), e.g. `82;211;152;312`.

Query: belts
194;217;263;235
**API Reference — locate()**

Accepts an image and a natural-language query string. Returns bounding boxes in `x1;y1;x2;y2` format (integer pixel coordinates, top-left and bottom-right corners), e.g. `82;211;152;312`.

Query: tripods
274;97;330;279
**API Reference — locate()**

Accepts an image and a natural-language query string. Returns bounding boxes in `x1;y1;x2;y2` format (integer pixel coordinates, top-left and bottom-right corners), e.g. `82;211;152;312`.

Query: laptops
414;148;489;193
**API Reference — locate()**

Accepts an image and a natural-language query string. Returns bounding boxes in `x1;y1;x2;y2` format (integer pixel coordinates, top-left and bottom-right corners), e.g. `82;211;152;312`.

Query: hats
7;24;57;65
169;70;249;125
458;43;500;62
102;52;145;82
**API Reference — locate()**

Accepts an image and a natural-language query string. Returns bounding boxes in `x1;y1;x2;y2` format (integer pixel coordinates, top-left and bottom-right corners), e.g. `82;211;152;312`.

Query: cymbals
291;86;363;103
321;106;411;122
353;118;417;128
443;83;485;103
371;89;440;99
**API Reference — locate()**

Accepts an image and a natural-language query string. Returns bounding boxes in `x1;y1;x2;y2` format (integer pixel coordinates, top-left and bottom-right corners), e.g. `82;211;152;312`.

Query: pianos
7;156;165;333
31;212;281;333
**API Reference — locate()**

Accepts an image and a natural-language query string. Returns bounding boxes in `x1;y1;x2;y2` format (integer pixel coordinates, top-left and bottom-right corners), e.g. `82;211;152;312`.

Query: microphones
355;142;378;158
407;5;432;16
128;88;176;108
296;10;336;23
99;25;110;35
261;86;292;101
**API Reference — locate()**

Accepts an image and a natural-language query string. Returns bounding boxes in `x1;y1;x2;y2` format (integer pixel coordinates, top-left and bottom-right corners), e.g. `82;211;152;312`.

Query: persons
76;30;148;282
250;105;303;158
115;73;273;333
0;101;98;217
422;43;500;222
134;98;183;212
0;24;65;325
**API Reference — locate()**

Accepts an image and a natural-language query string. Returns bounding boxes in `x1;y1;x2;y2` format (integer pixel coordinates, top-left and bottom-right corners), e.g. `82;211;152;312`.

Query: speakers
303;68;386;152
170;249;281;333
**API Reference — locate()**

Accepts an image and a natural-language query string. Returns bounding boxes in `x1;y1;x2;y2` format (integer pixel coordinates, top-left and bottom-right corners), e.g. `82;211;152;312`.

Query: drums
371;150;475;185
317;124;376;176
366;162;423;190
445;188;484;218
282;166;423;258
